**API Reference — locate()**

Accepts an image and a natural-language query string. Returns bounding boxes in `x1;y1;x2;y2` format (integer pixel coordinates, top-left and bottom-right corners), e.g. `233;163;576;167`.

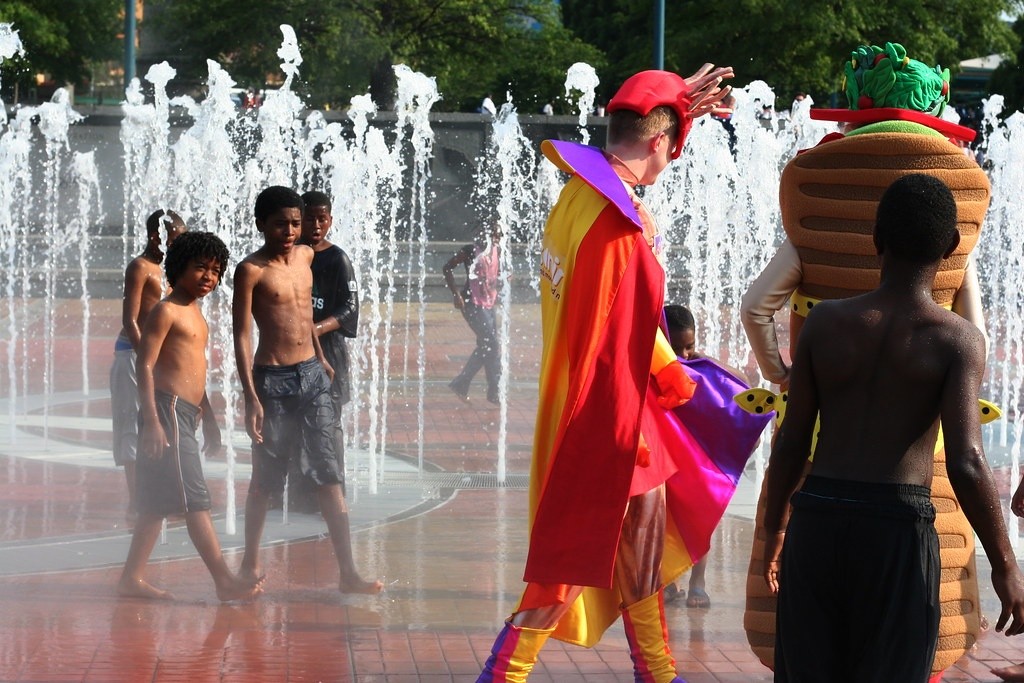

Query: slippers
662;583;684;605
687;588;708;608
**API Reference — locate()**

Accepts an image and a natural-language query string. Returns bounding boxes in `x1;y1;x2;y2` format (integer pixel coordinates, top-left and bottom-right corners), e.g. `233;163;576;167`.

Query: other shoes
451;385;470;403
487;396;501;406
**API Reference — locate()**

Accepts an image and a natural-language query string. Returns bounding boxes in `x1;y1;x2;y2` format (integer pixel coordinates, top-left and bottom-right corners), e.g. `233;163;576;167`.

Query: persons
109;209;200;531
232;188;382;595
116;231;268;603
662;303;760;608
444;225;510;408
763;175;1023;682
267;190;359;513
741;54;990;683
474;70;686;683
